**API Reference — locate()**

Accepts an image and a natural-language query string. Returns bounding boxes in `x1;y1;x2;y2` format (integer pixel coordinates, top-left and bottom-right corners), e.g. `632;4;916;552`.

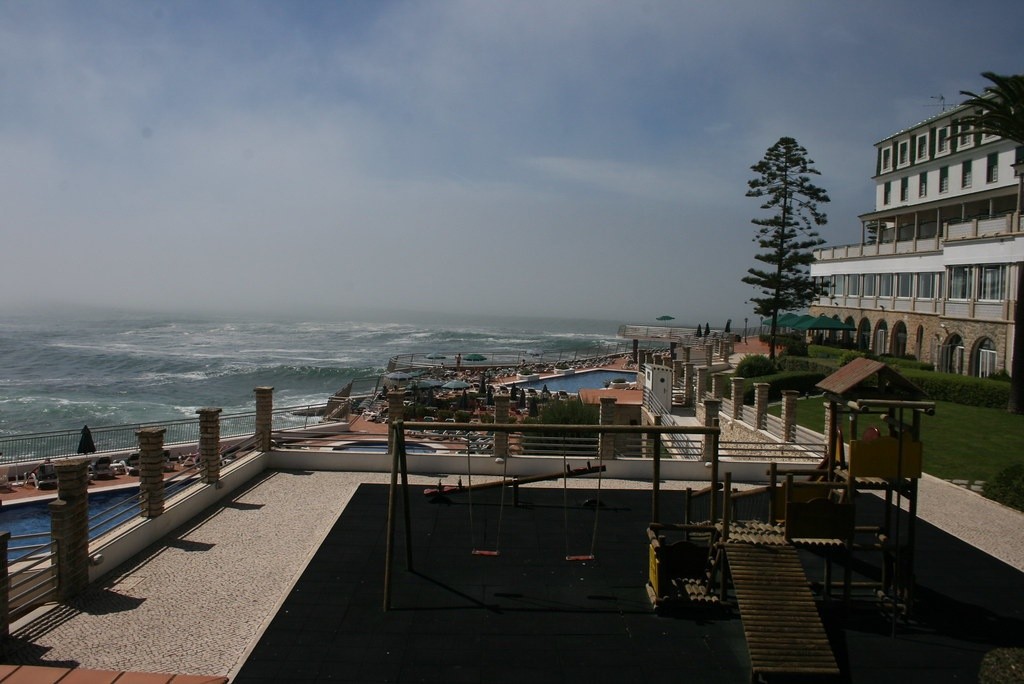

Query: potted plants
554;363;575;374
517;367;541;381
611;377;629;389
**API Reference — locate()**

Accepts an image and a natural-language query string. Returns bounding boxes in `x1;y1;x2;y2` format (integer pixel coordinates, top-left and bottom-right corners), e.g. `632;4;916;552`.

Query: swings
466;432;509;556
561;430;604;561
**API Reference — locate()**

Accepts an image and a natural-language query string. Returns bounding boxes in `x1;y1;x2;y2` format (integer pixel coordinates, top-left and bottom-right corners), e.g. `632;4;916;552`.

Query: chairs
410;415;495;442
22;444;232;490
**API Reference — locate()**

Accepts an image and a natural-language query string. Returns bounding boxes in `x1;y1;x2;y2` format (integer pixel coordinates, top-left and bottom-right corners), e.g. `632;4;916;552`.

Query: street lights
760;316;764;334
745;318;748;343
